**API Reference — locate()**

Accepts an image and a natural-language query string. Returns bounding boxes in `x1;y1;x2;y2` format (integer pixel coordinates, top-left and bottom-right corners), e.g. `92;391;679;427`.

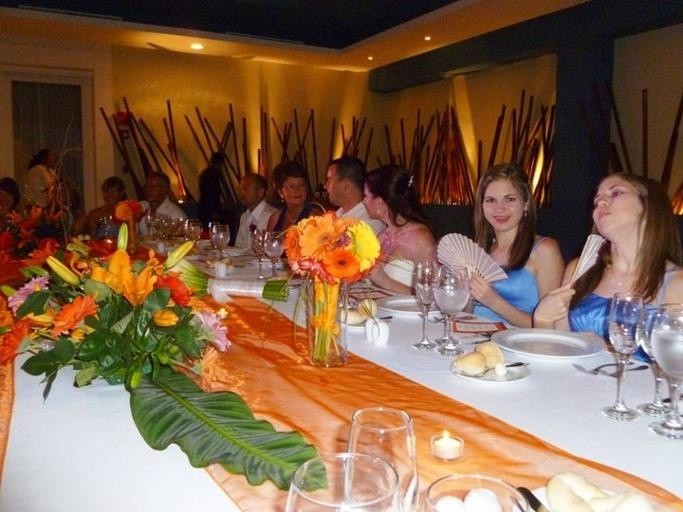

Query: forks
570;360;648;382
458;360;527;379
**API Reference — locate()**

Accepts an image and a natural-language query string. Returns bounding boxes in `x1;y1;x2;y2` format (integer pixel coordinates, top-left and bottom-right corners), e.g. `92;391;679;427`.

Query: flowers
278;215;384;289
3;223;329;494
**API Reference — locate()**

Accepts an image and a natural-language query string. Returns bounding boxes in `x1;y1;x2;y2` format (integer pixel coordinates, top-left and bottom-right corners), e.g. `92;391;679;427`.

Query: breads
476;343;501;368
357;299;378;318
454;352;485;376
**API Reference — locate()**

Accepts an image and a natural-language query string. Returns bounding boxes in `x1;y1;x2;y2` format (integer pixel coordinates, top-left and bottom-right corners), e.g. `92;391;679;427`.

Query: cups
429;435;465;462
344;406;420;511
284;451;399;512
424;473;529;511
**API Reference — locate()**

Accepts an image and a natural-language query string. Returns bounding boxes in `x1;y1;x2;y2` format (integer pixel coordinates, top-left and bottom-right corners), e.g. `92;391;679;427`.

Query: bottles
364;315;391;347
213;260;226;278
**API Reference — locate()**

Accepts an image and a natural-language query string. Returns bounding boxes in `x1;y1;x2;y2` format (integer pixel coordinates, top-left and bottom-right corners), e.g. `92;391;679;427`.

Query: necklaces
605;272;639;288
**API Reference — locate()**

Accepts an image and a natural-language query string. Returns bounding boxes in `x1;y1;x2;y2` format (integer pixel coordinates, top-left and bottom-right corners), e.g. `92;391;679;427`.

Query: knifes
518;486;550;511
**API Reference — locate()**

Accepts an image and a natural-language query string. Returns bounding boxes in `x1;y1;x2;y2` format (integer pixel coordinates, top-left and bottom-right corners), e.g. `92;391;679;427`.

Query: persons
532;171;683;362
233;158;438;298
197;149;234;232
0;150;189;238
439;162;561;335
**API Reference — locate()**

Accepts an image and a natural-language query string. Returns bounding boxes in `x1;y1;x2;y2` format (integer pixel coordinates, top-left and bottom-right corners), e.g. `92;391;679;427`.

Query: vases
300;278;347;369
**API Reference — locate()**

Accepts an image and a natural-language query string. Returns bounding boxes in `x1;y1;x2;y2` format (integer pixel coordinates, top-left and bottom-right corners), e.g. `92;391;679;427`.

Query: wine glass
144;209;230;259
411;257;472;357
596;290;683;440
251;230;285;281
101;215;110;236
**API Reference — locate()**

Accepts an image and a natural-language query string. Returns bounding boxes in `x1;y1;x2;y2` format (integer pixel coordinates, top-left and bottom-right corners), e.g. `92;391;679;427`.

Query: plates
513;484;618;512
448;360;528;384
490;327;600;360
374;296;442;318
337;319;391;333
